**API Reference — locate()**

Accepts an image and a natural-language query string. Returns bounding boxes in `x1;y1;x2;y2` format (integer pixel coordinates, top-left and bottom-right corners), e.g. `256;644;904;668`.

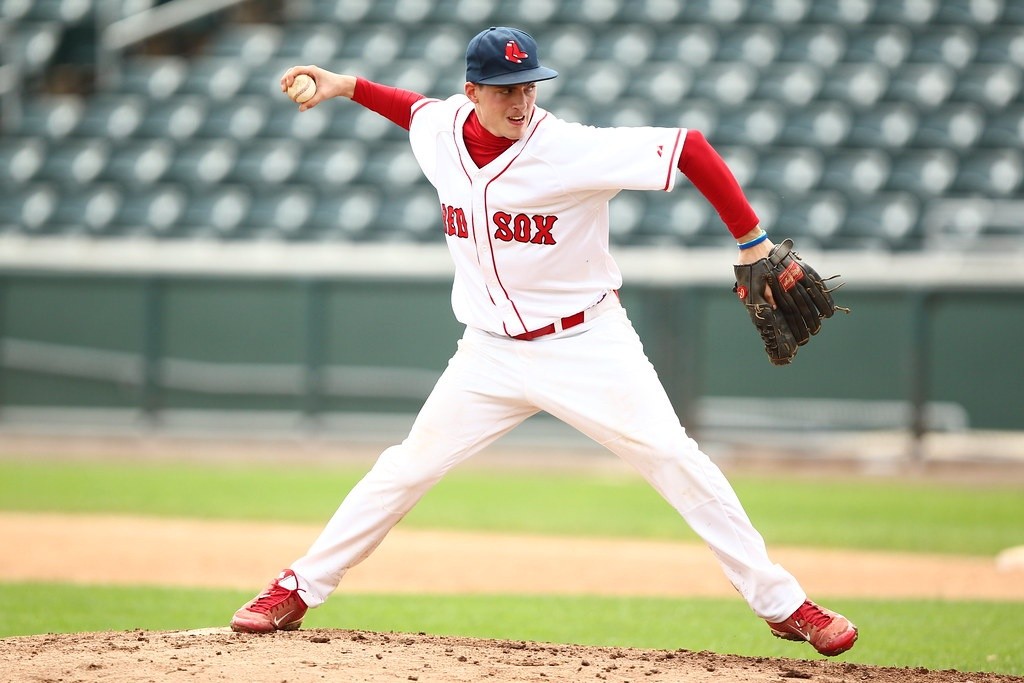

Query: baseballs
286;75;316;104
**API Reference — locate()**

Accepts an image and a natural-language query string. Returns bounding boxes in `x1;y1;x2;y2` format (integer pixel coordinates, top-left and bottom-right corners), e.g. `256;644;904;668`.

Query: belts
512;312;584;340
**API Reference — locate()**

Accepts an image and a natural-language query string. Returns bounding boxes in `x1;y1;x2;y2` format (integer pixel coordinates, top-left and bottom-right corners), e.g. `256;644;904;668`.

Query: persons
230;26;859;658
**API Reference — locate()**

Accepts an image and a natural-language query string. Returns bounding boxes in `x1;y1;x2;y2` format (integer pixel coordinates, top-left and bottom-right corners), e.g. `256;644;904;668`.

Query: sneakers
229;568;308;632
765;597;859;656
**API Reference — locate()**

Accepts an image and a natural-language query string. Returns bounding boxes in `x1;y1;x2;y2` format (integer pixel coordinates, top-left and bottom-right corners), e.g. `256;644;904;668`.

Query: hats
466;26;559;86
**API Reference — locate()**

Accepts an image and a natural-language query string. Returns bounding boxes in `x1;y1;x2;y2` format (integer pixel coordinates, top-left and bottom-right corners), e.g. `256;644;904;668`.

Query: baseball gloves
731;238;850;366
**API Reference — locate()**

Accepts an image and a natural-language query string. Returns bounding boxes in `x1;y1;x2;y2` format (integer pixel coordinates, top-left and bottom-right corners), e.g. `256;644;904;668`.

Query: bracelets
737;229;768;251
